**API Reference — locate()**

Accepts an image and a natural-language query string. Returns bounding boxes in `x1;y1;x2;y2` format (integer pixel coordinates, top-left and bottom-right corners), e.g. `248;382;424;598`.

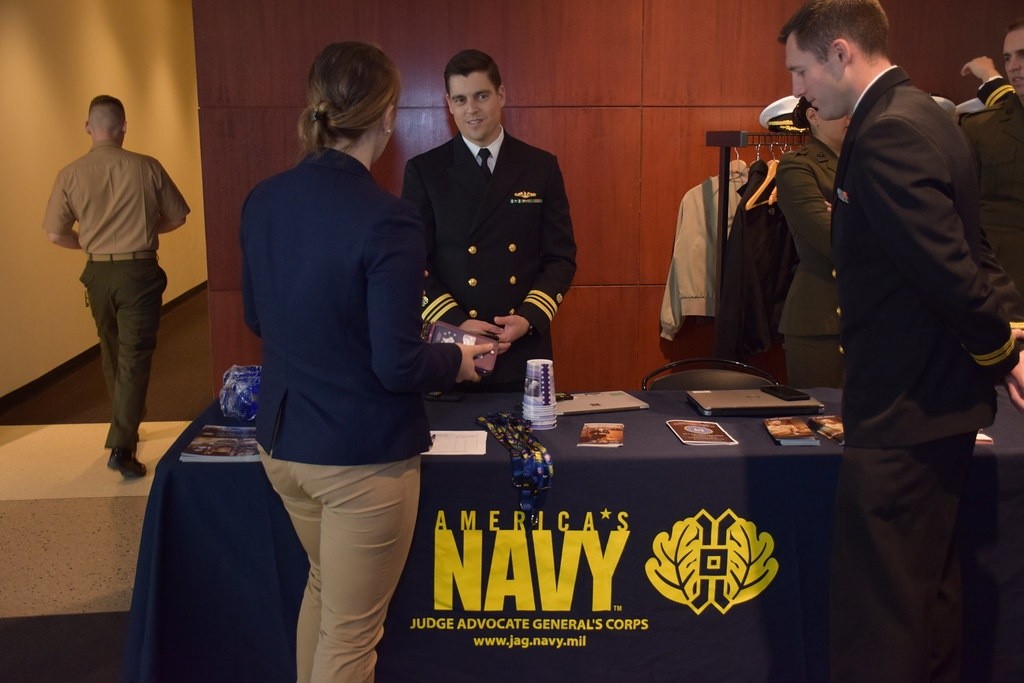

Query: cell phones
431;325;499;374
760;385;809;402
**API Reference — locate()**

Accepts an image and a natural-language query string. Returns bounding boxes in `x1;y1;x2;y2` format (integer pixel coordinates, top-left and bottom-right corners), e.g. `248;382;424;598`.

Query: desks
127;388;1024;683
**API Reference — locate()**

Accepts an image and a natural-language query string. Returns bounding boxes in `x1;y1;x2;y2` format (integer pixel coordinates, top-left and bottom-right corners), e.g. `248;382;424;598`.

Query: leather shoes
108;448;147;477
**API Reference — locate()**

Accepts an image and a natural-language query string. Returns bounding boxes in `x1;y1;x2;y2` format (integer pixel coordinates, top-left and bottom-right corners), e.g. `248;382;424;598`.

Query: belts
87;252;156;261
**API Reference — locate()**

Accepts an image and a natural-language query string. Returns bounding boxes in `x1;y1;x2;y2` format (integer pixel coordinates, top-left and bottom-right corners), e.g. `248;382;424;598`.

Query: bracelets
527;323;533;335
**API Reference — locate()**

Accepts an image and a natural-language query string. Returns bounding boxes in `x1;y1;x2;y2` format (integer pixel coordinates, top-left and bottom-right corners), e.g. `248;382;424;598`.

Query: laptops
686;389;825;417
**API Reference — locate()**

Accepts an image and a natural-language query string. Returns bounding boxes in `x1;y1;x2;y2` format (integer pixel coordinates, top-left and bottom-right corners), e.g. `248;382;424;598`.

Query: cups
522;359;557;430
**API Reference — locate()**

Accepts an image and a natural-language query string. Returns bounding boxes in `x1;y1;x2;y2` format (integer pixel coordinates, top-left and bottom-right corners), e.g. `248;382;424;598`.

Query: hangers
731;141;802;211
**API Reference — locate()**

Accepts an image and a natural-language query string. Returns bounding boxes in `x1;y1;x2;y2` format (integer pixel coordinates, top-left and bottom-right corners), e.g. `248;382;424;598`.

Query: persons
400;48;578;392
776;0;1024;683
240;41;494;683
42;95;191;478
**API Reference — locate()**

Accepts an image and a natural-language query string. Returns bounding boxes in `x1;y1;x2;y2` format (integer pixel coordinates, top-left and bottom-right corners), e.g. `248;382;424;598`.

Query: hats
759;95;809;133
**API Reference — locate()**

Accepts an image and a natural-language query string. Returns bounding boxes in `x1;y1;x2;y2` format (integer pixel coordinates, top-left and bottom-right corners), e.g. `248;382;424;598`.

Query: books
666;420;739;446
763;414;993;448
181;424;262;463
575;422;625;447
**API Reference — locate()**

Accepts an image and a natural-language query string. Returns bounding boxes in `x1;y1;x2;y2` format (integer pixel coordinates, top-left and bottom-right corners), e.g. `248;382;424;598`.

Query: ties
479;148;492;180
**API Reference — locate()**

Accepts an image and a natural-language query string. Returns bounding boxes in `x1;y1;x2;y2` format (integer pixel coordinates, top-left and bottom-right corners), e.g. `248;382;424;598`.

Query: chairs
641;358;782;391
